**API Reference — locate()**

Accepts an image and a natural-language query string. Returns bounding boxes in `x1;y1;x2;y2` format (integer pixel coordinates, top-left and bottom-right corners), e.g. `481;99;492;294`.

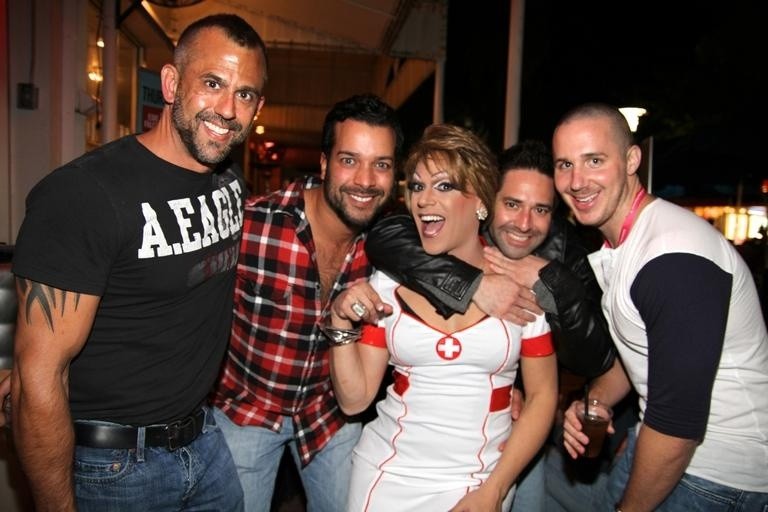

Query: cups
575;399;614;457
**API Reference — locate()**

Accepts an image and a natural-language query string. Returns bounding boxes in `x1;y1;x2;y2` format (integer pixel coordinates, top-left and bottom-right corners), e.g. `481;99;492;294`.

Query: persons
552;104;768;511
6;12;269;511
215;95;633;512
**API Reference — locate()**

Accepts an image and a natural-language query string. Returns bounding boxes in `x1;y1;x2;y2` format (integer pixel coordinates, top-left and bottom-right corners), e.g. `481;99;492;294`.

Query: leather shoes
73;410;205;453
